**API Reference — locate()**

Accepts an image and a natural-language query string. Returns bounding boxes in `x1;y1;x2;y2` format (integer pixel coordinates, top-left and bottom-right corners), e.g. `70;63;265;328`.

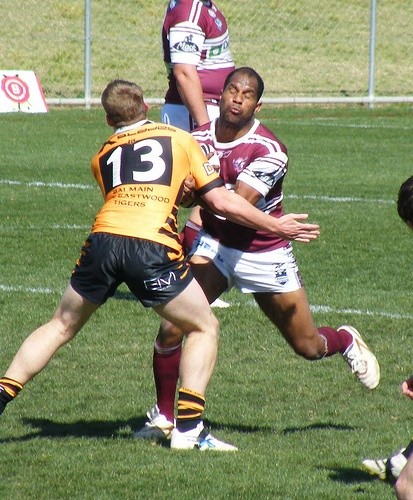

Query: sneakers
337;326;380;390
133;404;173;447
362;447;407;479
170;421;238;452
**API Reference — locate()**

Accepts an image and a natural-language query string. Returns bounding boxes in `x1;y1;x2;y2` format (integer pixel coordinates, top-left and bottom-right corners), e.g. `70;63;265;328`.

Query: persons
161;0;235;308
133;66;380;440
0;79;320;452
362;174;413;500
